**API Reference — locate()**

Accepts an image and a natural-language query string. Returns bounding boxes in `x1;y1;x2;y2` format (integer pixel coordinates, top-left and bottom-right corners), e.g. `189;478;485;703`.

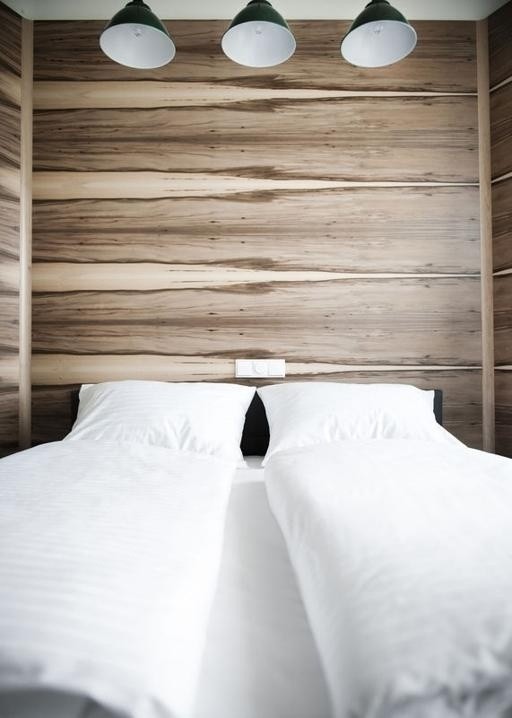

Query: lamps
219;0;297;68
98;0;177;70
338;0;418;68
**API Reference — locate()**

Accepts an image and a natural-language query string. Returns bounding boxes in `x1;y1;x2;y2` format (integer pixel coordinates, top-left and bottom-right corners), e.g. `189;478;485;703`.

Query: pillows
256;383;469;467
60;379;258;468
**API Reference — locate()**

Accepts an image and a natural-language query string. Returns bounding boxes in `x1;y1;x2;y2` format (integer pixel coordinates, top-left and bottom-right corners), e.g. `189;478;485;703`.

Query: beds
0;377;512;718
